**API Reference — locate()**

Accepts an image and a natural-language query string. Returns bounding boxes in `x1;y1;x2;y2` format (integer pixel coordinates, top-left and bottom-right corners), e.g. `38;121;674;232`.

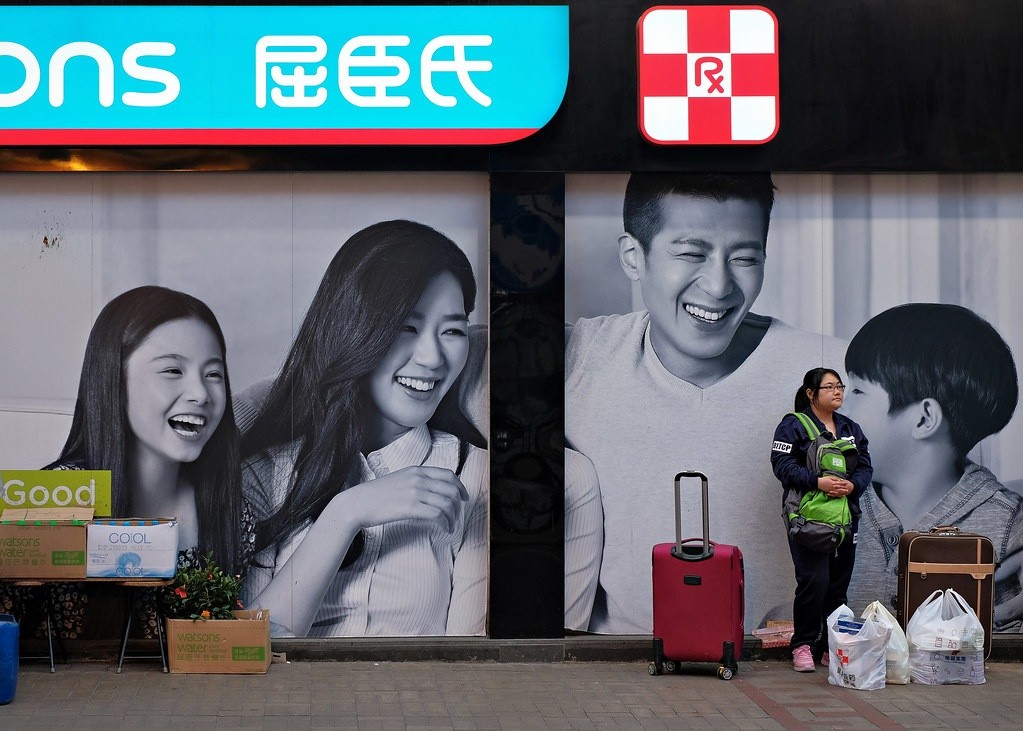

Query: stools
11;580;63;674
119;581;177;673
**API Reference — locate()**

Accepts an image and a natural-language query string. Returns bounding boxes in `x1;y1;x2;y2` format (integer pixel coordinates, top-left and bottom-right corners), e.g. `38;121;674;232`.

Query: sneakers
821;652;829;666
792;644;817;672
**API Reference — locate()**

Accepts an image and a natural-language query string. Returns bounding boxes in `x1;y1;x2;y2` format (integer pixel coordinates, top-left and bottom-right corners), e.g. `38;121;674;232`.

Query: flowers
163;553;247;621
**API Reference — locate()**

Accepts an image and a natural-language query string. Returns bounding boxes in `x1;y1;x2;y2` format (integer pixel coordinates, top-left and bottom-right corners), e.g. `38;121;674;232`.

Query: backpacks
780;411;859;547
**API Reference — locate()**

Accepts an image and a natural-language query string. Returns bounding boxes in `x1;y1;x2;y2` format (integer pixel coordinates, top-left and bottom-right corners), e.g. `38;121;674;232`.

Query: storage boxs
0;507;94;578
85;516;179;578
833;612;892;635
167;609;270;675
763;621;793;649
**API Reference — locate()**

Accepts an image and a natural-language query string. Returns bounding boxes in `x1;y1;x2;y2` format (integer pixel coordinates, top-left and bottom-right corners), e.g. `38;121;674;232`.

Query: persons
751;304;1023;634
238;218;602;637
0;285;241;637
769;367;874;673
230;173;849;634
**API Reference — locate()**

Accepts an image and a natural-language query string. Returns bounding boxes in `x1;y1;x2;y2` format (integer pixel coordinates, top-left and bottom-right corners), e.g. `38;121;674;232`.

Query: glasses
814;384;846;392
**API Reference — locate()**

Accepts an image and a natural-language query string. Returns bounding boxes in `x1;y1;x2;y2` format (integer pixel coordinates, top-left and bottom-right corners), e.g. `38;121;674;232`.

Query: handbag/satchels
860;600;909;685
906;588;987;685
826;603;893;691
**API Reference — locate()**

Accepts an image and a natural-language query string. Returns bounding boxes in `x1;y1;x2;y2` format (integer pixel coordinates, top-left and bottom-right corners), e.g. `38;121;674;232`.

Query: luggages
648;469;745;681
896;525;996;666
